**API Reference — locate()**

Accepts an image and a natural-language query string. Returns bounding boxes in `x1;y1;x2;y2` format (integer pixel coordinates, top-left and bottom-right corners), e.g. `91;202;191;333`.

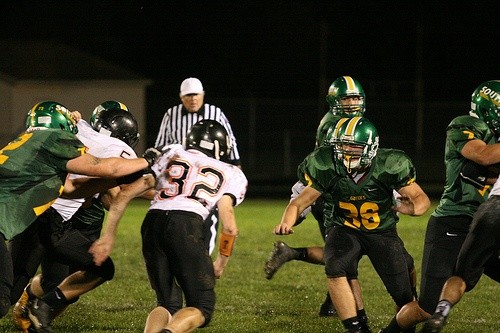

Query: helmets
330;116;379;173
90;100;129;128
26;101;78;135
326;76;365;116
94;108;140;150
186;118;230;163
469;80;500;133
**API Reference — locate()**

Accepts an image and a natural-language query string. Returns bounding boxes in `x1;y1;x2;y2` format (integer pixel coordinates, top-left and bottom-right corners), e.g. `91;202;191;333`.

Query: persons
0;78;248;333
263;76;500;333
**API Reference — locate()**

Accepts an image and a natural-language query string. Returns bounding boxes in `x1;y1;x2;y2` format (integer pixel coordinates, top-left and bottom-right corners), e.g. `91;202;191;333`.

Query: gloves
137;145;171;167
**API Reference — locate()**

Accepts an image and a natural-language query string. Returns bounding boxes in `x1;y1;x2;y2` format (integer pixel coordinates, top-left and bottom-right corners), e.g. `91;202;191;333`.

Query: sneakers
13;283;54;333
264;241;294;280
419;315;445;333
320;291;338;316
342;326;374;333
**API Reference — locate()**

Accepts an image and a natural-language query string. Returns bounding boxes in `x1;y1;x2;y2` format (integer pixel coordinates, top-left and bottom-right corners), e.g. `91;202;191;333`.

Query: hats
180;78;203;97
